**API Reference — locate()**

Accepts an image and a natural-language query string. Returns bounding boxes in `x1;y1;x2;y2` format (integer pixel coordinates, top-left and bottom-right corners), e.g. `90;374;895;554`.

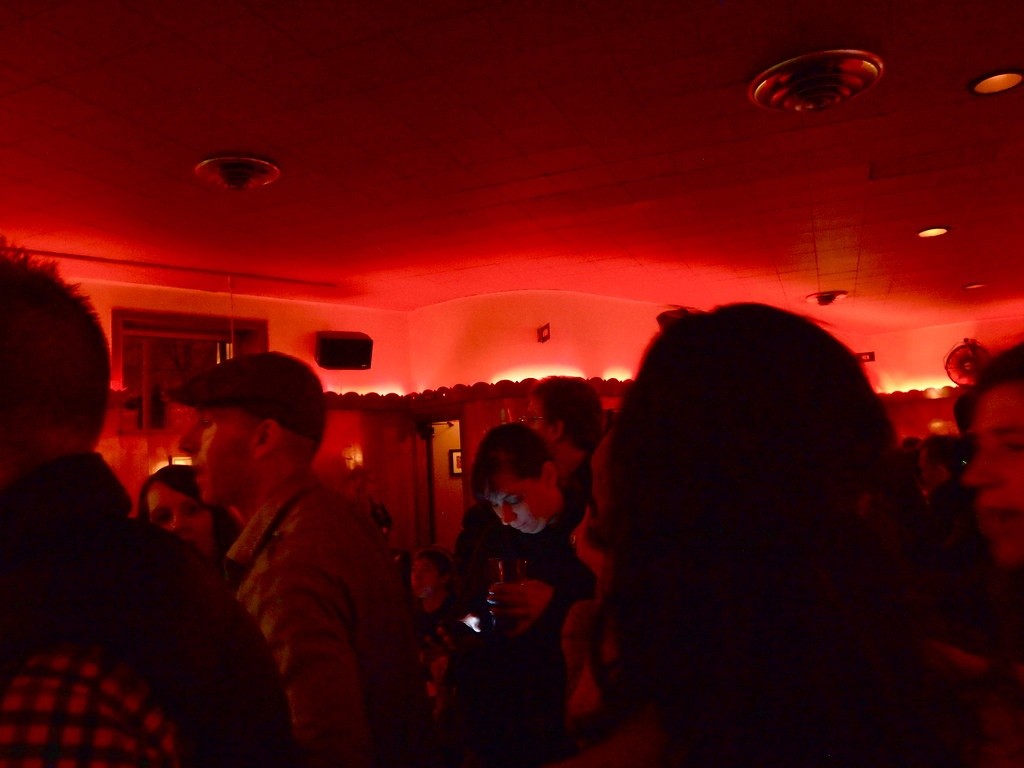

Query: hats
168;351;324;438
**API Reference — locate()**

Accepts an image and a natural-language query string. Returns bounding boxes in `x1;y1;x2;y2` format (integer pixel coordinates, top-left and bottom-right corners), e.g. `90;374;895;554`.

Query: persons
0;240;297;768
952;341;1024;568
138;464;246;599
370;375;613;768
918;433;990;576
566;302;1024;768
165;351;439;768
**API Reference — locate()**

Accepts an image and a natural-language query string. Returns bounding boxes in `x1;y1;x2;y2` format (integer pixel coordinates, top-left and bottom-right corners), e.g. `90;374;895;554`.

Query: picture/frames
450;449;462;475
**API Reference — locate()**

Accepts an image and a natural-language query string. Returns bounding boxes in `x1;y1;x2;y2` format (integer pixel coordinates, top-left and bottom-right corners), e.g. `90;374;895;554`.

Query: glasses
520;416;543;426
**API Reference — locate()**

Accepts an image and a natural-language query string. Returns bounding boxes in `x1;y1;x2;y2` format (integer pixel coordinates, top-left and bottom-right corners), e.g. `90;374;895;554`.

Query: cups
489;557;527;635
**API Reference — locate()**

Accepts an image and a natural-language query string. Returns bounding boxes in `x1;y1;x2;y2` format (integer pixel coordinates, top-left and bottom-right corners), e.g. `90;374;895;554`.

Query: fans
945;338;992;387
